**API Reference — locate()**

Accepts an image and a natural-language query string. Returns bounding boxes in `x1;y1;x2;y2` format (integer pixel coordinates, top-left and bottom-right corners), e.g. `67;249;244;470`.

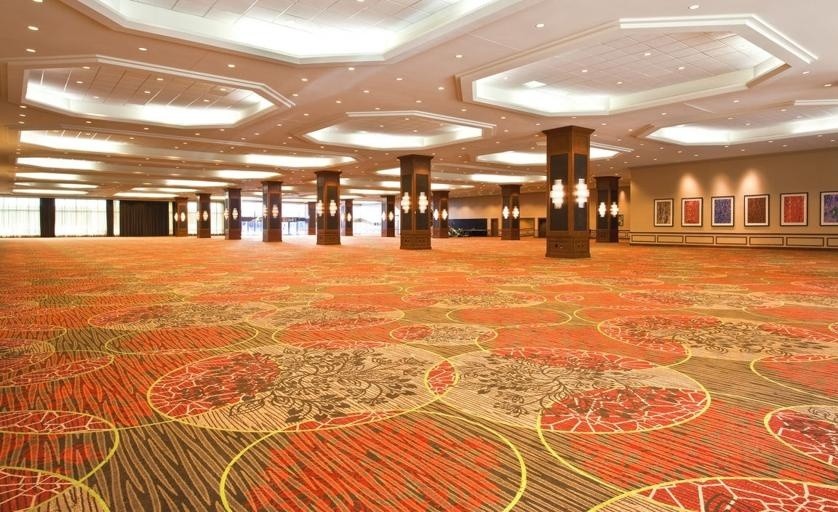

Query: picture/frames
819;191;837;226
710;195;734;227
779;192;808;227
680;197;703;227
743;194;768;227
653;197;673;227
